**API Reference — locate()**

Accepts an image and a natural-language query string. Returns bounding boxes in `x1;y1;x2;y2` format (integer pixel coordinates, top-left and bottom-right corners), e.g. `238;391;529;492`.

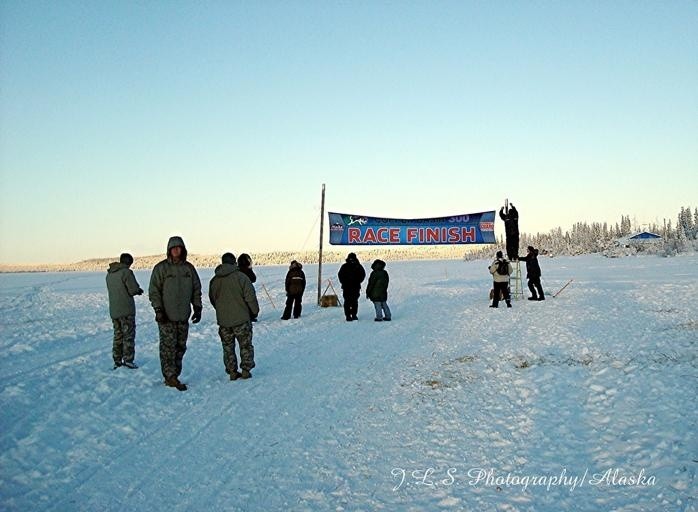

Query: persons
149;236;202;391
499;203;519;262
106;253;144;369
281;260;306;320
238;253;257;323
338;253;365;322
209;253;259;380
517;246;545;301
366;260;391;321
489;251;513;308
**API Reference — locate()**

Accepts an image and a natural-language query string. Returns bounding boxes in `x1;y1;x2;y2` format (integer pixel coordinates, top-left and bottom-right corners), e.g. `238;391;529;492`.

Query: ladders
508;258;524;301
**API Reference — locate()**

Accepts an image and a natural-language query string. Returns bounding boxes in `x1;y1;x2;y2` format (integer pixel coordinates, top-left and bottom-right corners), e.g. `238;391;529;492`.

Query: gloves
154;312;166;323
191;309;202;325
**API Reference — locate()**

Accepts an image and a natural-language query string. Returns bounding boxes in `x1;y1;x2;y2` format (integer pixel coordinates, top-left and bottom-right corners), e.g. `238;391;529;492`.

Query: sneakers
240;369;252;378
229;371;242;381
113;361;140;370
164;376;188;392
340;316;392;322
